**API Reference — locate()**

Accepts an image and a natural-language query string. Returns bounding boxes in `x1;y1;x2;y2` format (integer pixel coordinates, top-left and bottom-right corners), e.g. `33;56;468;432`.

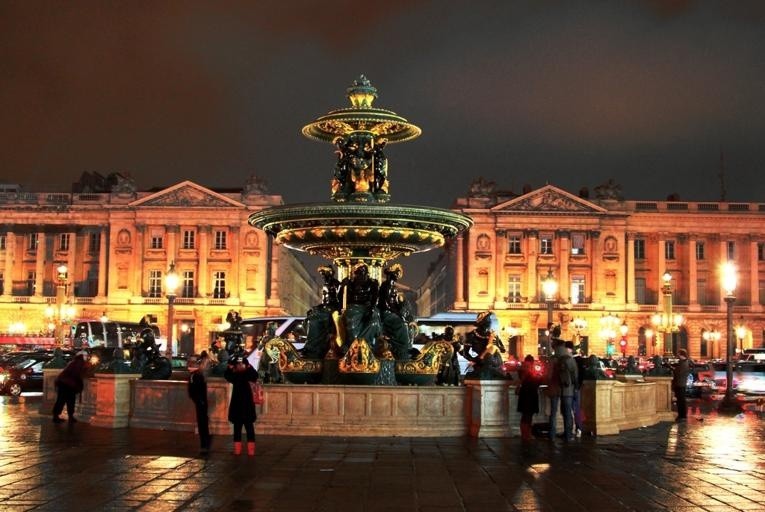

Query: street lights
570;315;587;358
162;260;182;360
661;268;674;360
540;265;559;357
53;260;68;345
620;321;628;358
720;261;737;400
600;312;621;358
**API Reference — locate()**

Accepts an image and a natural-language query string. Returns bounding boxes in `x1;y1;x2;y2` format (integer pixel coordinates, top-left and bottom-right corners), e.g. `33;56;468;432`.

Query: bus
229;312;500;375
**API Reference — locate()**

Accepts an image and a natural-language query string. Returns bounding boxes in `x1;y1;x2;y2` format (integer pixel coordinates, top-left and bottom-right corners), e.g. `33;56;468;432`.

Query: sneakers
575;429;582;437
548;434;574;443
53;414;64;423
68;416;77;424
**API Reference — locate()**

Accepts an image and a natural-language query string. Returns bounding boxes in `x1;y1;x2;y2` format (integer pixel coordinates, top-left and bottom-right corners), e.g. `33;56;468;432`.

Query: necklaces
473;328;493;340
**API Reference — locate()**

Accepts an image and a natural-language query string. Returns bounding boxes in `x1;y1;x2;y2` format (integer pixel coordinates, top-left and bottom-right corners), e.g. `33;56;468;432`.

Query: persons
544;338;580;444
431;327;476;385
51;350;95;424
225;355;259;458
258;321;285;384
668;349;691;423
186;359;213;449
460;312;511;381
517;355;542;446
301;263;419;363
562;341;586;441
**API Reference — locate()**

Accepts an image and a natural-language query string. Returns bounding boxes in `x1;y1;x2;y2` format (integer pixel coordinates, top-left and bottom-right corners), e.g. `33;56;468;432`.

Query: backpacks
553;354;572;387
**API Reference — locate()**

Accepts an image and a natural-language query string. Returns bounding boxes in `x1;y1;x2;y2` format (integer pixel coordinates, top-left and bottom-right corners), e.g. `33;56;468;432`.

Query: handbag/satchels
515;383;521;395
249;381;263;405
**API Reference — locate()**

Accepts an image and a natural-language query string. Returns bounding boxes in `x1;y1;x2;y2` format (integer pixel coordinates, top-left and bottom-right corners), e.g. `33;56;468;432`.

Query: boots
233;441;241;456
521;423;536;440
248;443;255;455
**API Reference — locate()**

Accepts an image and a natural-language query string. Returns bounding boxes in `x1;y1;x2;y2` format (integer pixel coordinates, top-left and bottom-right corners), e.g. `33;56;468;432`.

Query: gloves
228;355;249;367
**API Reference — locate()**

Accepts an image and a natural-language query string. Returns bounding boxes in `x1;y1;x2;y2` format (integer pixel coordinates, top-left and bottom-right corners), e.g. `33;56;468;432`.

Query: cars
713;348;765;396
0;351;75;396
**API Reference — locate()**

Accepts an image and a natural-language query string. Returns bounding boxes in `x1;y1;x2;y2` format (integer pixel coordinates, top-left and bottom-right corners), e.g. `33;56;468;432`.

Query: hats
77;351;87;356
